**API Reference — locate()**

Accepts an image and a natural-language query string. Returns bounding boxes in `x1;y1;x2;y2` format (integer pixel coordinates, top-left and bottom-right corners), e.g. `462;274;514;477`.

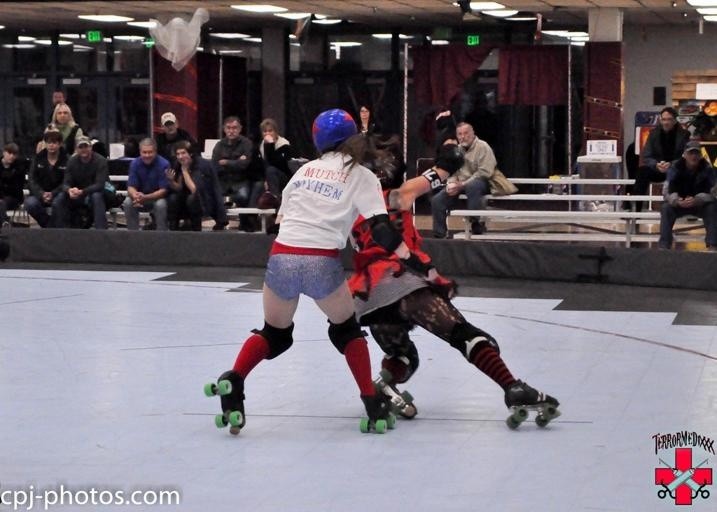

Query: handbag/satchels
488;169;518;195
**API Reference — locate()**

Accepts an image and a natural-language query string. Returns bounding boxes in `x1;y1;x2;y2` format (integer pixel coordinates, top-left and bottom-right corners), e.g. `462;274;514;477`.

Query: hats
161;112;176;125
686;141;700;152
77;137;90;147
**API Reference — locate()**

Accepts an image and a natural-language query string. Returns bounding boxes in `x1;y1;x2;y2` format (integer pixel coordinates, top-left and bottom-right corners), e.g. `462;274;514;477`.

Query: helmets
312;109;403;189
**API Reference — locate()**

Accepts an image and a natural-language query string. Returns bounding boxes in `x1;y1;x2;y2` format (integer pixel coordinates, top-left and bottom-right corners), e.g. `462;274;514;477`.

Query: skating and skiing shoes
204;371;244;435
505;379;559;428
360;370;416;434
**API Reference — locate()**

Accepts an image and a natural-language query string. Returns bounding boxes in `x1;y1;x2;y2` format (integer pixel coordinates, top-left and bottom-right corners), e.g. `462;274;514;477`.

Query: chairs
409;157;436;217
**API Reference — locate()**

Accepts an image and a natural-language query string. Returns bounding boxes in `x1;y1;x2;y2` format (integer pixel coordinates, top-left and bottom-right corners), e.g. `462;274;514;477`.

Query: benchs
0;139;281;237
441;171;717;250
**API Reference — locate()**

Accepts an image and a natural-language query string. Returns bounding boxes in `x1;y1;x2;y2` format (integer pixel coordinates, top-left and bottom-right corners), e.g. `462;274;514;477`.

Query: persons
337;131;560;420
41;104;81;155
351;106;380;135
51;137;109;229
163;140;214;231
429;120;496;239
247;118;291;234
23;131;68;228
215;105;393;424
691;100;716;163
659;139;716;251
630;108;691;212
211;116;255;231
151;112;197;155
119;136;170;232
0;142;32;228
